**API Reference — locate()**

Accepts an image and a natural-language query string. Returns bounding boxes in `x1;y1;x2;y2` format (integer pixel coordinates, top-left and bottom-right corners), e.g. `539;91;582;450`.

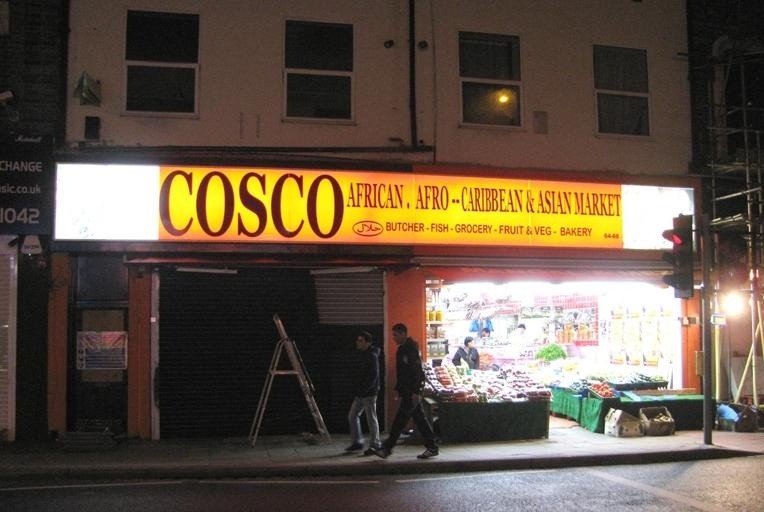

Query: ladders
247;313;333;448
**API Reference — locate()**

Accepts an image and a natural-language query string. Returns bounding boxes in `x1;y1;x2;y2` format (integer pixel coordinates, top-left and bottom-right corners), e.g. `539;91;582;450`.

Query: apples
592;383;613;397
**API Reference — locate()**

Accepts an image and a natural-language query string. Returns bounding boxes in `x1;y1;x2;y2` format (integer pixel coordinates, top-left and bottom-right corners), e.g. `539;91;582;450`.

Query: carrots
436;365;467;403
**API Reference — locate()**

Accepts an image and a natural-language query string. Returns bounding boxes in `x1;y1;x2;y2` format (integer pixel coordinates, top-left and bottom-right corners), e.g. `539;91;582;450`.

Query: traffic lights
671;229;683;289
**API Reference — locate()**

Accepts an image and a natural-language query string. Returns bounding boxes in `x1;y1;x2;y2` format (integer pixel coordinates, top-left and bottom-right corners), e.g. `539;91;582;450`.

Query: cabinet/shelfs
426;321;715;443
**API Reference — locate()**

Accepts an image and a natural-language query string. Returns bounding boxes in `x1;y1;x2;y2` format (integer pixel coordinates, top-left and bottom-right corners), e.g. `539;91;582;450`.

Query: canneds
425;311;442;322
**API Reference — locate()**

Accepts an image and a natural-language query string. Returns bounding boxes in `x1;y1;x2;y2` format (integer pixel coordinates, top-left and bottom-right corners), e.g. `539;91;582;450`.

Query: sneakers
417;446;439;458
345;439;378;458
368;445;388;459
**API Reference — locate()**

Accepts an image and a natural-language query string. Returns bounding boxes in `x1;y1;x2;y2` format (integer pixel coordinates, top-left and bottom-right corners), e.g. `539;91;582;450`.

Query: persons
449;335;481;371
715;399;740;431
368;323;439;458
481;327;490;344
343;330;382;456
511;323;525;337
